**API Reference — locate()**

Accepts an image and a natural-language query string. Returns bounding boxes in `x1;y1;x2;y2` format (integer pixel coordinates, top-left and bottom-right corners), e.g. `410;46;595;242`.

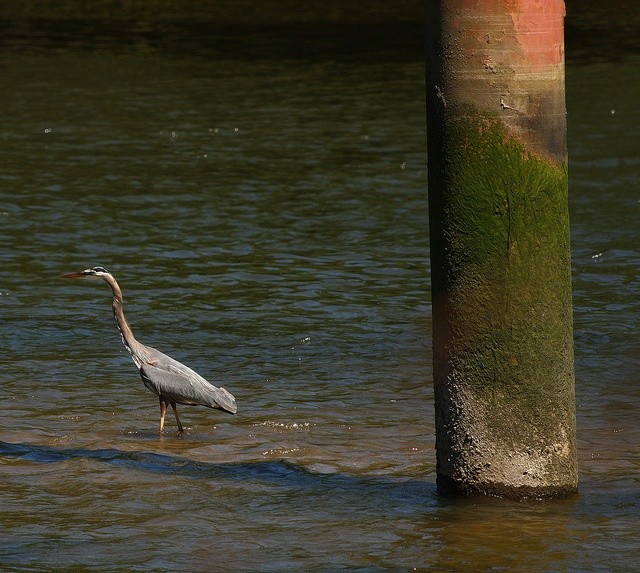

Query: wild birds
55;264;240;437
